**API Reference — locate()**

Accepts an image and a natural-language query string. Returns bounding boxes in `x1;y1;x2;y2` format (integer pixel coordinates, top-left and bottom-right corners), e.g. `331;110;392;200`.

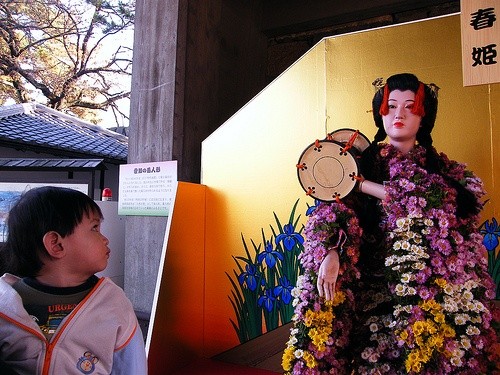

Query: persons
0;186;148;375
283;70;496;375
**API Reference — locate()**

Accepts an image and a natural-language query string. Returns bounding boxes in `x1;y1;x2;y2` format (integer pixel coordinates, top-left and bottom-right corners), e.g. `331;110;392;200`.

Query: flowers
475;213;500;250
233;198;322;310
280;143;500;374
